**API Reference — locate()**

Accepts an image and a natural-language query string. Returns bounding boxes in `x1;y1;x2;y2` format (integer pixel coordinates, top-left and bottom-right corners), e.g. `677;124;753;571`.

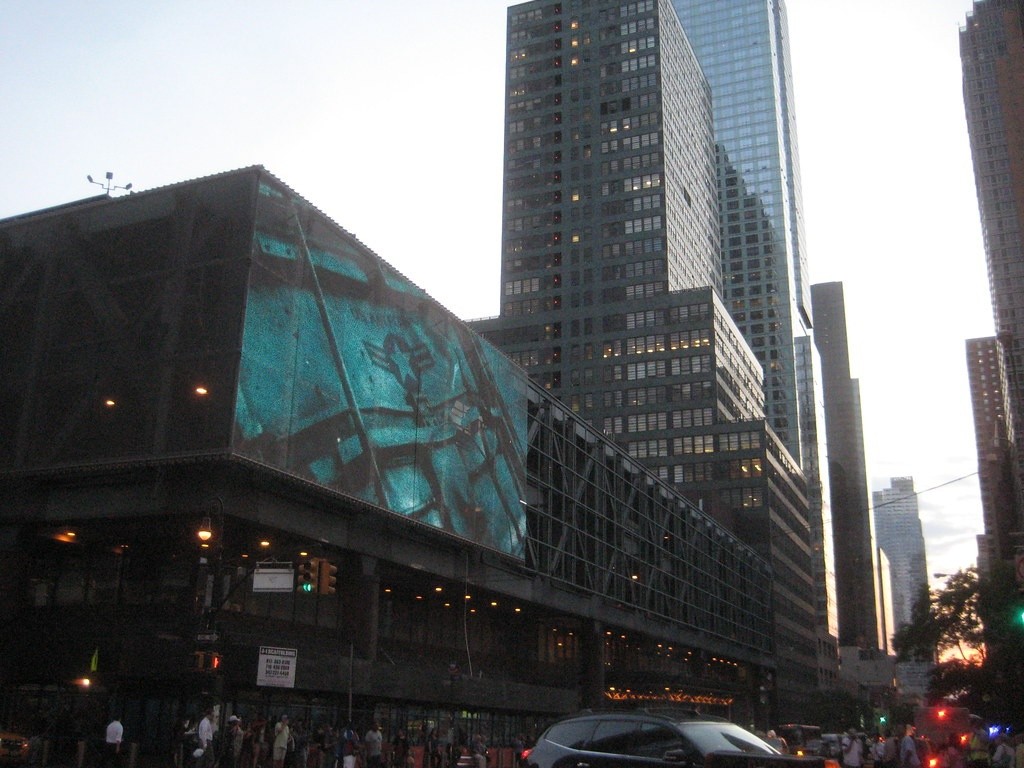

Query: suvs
518;707;841;768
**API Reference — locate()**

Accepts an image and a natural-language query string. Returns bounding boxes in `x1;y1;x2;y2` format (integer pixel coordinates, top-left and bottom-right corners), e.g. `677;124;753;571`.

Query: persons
769;724;1024;768
194;708;216;768
104;714;123;768
221;713;526;768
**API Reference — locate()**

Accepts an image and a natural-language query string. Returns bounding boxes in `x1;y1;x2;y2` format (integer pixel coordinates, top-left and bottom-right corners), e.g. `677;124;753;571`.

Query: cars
821;734;850;755
0;728;29;764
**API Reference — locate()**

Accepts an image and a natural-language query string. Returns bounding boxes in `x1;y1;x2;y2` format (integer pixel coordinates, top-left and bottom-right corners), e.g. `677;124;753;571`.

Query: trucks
913;707;989;741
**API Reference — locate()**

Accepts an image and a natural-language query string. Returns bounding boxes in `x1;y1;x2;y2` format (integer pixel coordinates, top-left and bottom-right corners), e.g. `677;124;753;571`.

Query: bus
779;724;821;753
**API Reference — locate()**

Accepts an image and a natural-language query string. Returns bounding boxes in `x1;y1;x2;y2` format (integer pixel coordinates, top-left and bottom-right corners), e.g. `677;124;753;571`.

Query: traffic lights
879;716;887;727
302;557;319;595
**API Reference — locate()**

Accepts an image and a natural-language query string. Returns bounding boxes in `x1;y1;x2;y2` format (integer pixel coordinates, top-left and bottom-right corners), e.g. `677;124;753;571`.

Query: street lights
51;526;124;604
195;496;225;708
